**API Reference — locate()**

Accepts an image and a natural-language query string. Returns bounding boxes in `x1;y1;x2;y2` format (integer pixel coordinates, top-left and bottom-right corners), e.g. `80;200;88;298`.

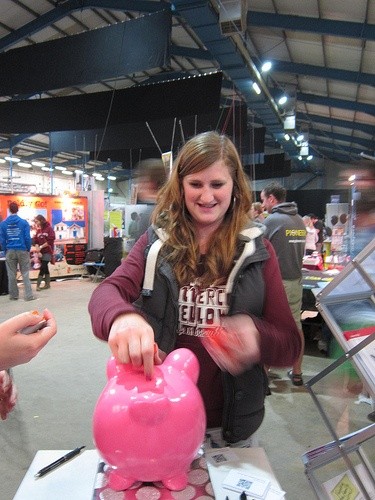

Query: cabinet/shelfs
302;235;375;500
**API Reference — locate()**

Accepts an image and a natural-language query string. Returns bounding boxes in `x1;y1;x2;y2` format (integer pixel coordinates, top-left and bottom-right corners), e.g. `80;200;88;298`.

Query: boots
35;274;50;291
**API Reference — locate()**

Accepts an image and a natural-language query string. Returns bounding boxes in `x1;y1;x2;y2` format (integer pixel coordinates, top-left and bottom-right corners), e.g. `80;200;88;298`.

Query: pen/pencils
224;491;248;500
33;445;86;477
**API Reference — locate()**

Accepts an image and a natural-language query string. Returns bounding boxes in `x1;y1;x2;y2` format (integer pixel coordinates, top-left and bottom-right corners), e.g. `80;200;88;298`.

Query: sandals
288;370;304;385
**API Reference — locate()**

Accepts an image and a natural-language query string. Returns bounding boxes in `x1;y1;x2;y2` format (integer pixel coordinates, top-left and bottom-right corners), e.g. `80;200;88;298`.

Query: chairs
83;256;106;282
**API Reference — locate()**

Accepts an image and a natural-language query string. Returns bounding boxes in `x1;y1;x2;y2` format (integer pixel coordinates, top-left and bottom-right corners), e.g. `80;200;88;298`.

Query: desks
11;448;287;500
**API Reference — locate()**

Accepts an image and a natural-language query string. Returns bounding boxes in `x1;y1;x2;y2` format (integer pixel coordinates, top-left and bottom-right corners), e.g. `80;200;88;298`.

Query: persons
88;130;303;443
250;180;331;385
0;202;56;301
0;306;58;420
129;212;141;242
130;159;168;250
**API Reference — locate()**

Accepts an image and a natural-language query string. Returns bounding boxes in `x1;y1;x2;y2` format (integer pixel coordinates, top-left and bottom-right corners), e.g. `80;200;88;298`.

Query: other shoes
25;296;37;301
9;298;18;300
